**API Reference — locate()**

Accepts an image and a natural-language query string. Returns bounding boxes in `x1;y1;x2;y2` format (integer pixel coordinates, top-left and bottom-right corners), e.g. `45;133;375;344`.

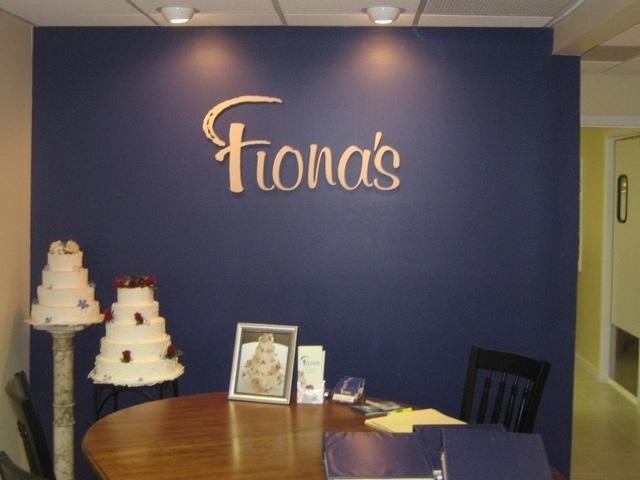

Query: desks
80;389;566;480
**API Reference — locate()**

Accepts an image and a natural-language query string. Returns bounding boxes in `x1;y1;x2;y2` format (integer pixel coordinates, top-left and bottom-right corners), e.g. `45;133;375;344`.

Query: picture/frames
226;322;297;402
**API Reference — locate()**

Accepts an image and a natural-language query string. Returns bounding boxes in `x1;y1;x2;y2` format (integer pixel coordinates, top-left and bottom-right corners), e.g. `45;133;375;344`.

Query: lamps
161;6;194;26
364;6;399;25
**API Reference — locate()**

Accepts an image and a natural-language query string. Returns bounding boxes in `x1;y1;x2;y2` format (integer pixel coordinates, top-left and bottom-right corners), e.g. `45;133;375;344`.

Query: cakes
242;334;285;394
30;238;100;327
88;284;180;384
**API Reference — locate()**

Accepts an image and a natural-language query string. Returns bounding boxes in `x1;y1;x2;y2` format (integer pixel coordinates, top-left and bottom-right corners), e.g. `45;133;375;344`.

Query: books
364;407;467;434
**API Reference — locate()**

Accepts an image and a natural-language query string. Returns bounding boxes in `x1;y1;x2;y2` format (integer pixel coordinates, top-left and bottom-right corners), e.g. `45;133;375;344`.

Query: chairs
4;370;58;479
462;347;552;434
